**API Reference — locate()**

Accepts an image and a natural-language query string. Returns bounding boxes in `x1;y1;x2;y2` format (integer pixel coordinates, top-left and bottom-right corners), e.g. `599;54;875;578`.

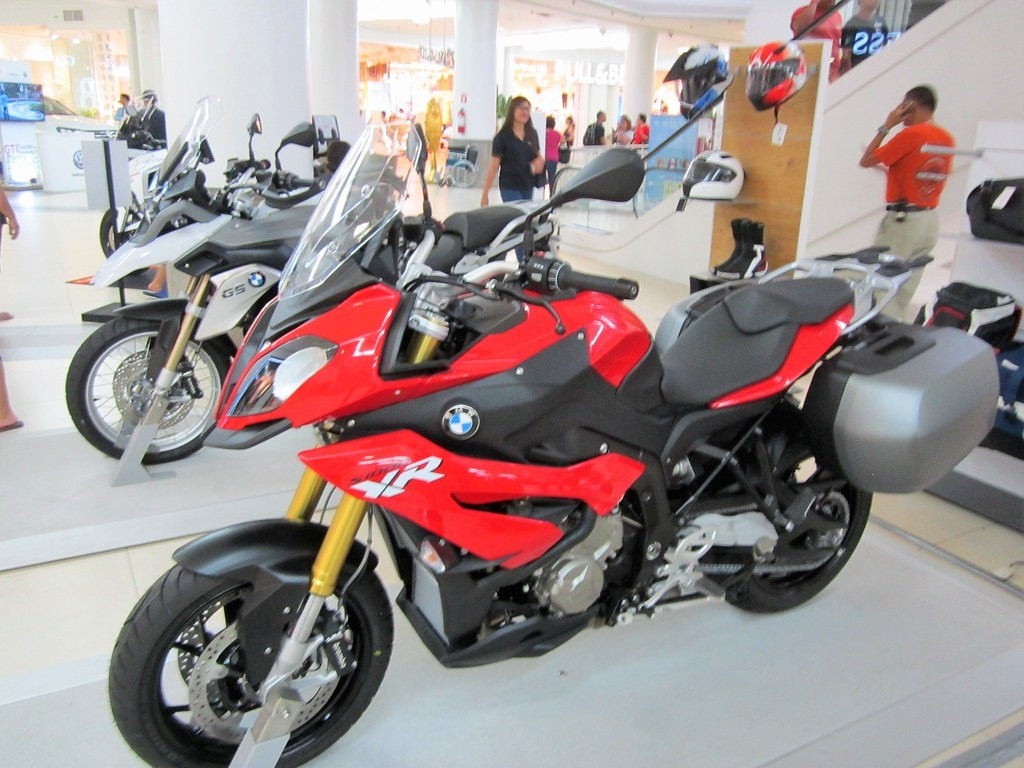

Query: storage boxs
652;276;763;362
817;326;1005;495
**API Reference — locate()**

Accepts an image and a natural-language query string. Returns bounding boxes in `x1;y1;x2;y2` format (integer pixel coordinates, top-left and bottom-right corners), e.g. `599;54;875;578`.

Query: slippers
143;288;168;298
0;421;23;433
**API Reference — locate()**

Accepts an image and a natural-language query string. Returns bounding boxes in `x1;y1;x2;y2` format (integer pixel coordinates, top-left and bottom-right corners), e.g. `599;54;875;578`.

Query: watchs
877;125;889;135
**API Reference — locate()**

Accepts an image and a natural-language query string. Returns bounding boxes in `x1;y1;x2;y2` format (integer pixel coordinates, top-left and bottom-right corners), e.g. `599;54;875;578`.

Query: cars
1;72;118;169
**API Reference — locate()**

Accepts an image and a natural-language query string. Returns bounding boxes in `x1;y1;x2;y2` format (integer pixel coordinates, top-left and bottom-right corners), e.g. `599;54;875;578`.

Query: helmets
682;149;744;200
663;43;729;120
746;40;804;111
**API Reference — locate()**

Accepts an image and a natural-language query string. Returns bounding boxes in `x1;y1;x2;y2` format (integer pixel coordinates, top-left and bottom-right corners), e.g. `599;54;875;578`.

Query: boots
711;218;768;279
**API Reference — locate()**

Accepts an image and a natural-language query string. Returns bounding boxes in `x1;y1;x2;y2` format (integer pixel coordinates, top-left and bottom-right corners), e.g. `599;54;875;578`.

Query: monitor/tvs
0;81;46;122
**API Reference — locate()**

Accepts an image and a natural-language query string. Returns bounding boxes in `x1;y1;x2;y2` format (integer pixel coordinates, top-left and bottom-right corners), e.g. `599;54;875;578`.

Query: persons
860;86;955;321
0;185;20;320
114;89;167;149
546;116;565;199
790;0;889;83
143;264;168;299
480;96;545;264
563;111;650;144
370;105;416;155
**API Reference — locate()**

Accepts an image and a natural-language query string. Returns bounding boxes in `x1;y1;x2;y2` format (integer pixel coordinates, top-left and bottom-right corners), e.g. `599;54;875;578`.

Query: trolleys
437;143;481;190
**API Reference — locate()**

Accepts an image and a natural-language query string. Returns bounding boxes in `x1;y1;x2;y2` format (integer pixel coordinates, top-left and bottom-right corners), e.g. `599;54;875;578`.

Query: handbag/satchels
910;281;1024;439
534;162;546;188
558;148;571;162
964;177;1024;246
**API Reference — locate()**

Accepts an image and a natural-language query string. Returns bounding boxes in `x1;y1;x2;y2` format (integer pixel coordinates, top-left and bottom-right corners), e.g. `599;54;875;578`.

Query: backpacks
582;124;601;145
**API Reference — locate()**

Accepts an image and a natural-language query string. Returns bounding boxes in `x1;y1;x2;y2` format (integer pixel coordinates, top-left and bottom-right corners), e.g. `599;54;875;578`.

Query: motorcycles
63;95;1002;768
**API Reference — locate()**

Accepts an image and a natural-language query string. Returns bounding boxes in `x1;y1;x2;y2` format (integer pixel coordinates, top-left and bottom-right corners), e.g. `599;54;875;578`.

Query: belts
885;203;934;211
